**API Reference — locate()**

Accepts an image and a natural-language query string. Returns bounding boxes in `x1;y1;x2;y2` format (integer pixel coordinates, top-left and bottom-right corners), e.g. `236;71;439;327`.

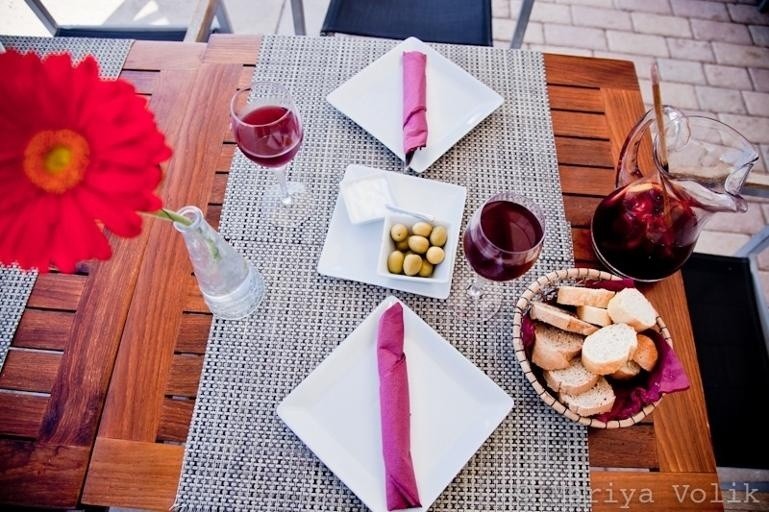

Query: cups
589;105;758;283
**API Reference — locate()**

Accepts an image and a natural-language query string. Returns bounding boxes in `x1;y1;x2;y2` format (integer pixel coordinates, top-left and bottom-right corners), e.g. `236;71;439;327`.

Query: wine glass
450;190;547;324
230;80;315;228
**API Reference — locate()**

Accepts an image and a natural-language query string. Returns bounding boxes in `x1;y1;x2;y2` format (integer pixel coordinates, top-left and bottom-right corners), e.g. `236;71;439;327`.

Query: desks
1;30;728;511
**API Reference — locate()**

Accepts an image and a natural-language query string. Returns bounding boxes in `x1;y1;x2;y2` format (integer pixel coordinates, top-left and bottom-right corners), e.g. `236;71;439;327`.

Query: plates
315;160;471;302
323;35;506;177
275;294;516;512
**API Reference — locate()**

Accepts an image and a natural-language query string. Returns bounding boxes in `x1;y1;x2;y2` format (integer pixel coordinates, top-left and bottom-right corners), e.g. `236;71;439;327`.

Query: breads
529;284;657;416
